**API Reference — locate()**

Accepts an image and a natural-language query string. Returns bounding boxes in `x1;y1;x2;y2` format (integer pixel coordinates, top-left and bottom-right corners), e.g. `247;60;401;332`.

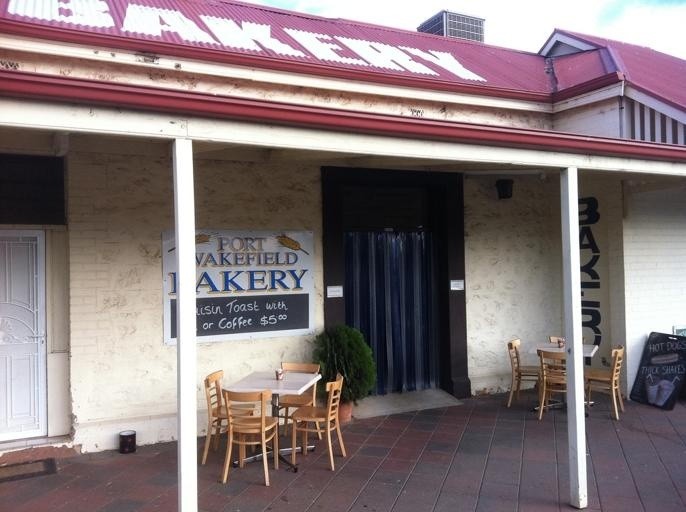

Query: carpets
0;458;58;482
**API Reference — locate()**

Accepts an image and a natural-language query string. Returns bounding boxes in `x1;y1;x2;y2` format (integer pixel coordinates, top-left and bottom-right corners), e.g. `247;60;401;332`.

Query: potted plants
306;322;378;423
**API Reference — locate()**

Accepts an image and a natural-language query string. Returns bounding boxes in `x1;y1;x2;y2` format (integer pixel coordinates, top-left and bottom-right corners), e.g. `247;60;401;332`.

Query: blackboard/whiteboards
164;292;317;346
629;332;686;410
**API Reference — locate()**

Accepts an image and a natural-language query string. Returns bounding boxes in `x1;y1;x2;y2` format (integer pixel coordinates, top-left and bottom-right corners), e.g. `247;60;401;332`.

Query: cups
645;377;660;404
655;380;675;408
275;368;284;380
558;337;566;348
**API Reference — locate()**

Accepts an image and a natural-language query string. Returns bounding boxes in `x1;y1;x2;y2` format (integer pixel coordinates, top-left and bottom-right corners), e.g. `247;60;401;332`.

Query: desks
225;370;322;473
528;341;599;418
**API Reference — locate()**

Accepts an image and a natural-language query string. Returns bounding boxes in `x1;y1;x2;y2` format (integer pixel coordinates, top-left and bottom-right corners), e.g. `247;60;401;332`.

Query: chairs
538;350;567;421
220;389;279;486
584;343;625;421
271;361;321;437
291;371;346;471
506;338;551;413
549;335;586;348
202;370;256;469
